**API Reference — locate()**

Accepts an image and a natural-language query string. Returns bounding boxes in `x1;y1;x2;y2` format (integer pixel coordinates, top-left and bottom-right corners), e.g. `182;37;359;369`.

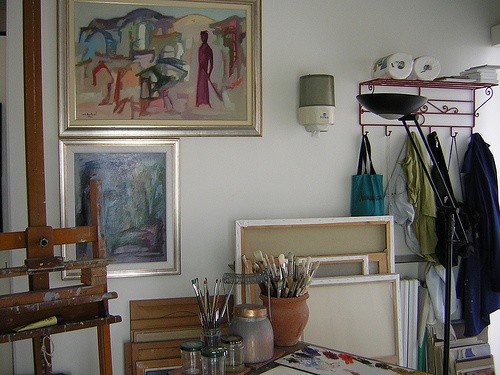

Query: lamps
355;92;481;375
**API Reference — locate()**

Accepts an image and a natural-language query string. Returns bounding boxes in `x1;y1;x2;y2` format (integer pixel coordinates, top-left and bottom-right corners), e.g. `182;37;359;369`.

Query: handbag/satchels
349;133;384;216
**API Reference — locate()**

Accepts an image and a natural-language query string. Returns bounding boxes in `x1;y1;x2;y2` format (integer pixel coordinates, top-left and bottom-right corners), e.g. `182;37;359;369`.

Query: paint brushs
243;251;321;297
190;277;236;328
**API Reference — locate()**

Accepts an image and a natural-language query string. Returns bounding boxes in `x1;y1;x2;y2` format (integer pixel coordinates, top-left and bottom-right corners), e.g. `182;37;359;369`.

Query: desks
170;341;429;375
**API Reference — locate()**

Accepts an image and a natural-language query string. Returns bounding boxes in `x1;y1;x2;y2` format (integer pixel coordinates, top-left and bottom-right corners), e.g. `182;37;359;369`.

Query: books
434;63;500;84
425;319;495;375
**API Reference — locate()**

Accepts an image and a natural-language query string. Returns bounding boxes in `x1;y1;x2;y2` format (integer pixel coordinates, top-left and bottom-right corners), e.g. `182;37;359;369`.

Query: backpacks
433;200;474;258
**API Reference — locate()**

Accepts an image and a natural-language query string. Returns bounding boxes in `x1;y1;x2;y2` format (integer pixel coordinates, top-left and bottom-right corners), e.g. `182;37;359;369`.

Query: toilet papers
374;53;413;79
406;56;441;80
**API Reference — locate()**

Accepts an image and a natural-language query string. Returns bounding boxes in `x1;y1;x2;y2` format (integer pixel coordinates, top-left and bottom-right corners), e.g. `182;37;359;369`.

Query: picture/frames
135;358;181;375
57;138;182;281
57;0;263;138
